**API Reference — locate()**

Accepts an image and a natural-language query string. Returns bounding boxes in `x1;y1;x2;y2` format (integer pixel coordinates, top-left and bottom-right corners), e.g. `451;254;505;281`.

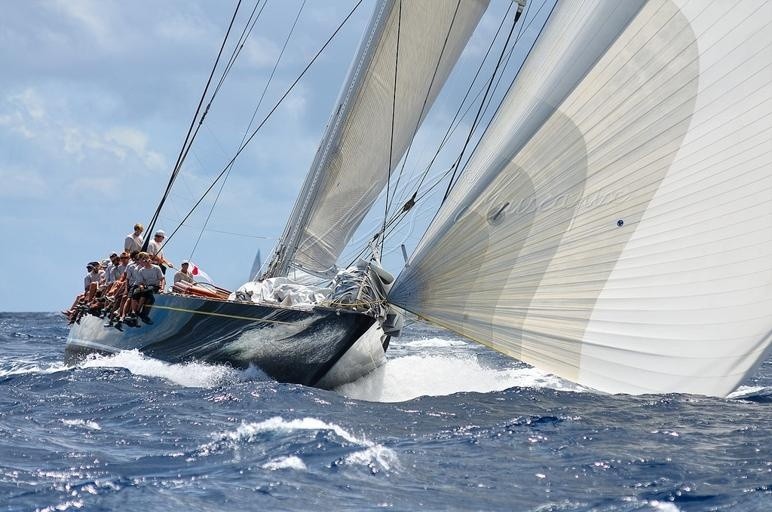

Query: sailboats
62;0;772;401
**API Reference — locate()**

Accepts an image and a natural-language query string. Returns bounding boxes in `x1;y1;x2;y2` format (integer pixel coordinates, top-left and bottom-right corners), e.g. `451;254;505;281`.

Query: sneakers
139;313;153;325
106;295;116;302
95;295;106;304
61;308;81;325
104;322;113;328
115;316;141;331
99;307;119;321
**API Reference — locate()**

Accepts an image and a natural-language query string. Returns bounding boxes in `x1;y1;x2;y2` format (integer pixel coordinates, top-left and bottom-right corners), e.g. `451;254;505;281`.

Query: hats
87;263;93;267
155;231;167;237
102;261;108;267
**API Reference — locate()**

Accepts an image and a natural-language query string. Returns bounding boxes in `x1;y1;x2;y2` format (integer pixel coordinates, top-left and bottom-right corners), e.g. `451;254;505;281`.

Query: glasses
120;256;128;259
143;260;151;263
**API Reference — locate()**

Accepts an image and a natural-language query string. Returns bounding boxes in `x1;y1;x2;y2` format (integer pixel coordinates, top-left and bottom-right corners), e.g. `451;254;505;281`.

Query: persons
148;229;174;276
173;259;194;284
60;251;167;332
125;223;146;254
